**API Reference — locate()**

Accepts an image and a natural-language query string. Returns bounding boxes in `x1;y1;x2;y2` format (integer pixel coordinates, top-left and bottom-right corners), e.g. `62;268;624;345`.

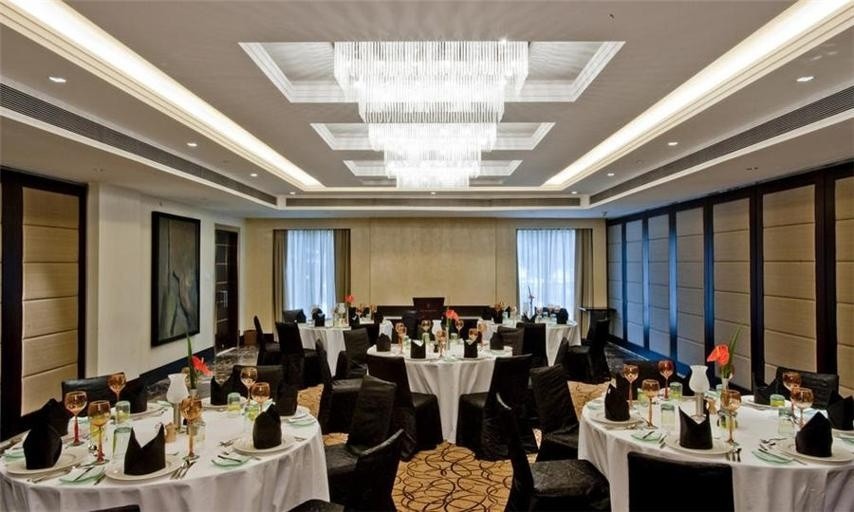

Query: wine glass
502;303;559;325
781;371;812;431
688;365;709;423
621;359;673;431
354;301;376;323
166;373;189;432
720;388;742;446
395;319;488;356
179;398;203;462
212;361;268;417
64;371;130;463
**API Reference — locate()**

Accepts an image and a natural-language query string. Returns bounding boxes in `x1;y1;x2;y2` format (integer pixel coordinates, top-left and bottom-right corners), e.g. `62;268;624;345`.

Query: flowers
342;295;353;306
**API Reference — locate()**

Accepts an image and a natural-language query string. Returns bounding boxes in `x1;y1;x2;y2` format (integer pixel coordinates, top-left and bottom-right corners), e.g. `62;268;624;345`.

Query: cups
669;383;681;404
660;403;674;431
770;395;785;410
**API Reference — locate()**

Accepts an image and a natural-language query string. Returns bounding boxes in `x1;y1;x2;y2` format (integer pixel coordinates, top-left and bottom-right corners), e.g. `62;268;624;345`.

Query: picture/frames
150;210;200;348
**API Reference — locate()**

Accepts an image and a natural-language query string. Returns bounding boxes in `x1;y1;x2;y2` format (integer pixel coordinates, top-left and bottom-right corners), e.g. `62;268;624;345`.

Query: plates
6;404;308;480
589;407;854;463
